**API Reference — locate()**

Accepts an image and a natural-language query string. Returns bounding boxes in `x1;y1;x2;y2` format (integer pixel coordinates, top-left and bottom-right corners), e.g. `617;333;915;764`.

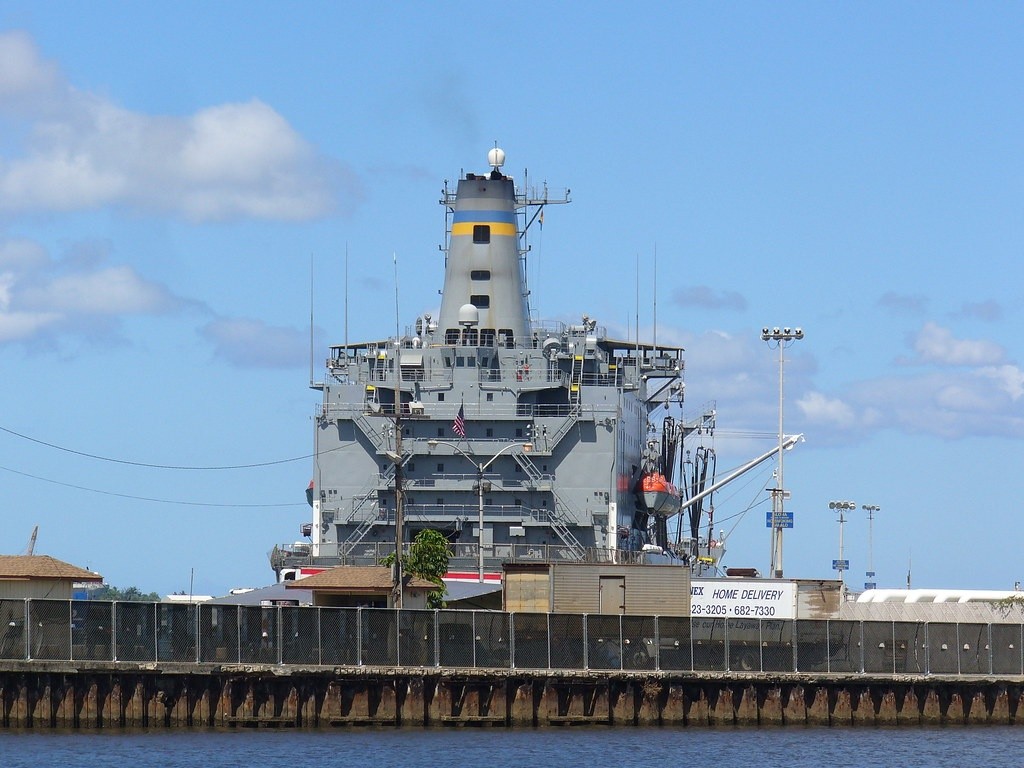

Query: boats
642;473;680;516
635;546;684;566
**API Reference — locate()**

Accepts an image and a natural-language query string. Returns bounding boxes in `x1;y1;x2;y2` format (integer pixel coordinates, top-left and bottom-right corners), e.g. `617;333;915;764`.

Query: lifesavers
709;540;716;549
647;509;655;516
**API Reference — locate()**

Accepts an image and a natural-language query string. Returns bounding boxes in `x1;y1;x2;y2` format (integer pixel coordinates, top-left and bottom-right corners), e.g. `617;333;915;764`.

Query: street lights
761;326;805;580
828;501;856;579
861;504;882;586
428;439;535;583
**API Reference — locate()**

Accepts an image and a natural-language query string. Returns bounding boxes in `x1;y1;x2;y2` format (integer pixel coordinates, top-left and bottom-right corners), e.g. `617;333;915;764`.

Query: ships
190;140;728;608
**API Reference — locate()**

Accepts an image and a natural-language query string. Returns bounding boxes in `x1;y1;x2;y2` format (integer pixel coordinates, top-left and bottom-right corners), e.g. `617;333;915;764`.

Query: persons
532;333;539;349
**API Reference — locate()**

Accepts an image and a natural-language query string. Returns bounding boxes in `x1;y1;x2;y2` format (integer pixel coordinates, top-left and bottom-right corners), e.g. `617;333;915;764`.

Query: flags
538;210;544;231
452;405;465;436
